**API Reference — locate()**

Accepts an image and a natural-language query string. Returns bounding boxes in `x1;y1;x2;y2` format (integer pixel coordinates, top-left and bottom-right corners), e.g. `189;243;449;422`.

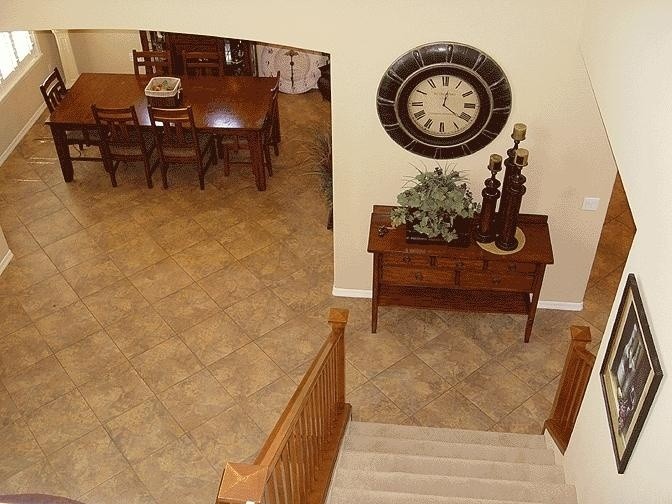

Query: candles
513;124;527;140
489;154;502;170
513;148;529;166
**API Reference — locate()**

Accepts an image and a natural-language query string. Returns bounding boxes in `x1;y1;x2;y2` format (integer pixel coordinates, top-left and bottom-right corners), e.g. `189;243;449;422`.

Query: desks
367;205;554;343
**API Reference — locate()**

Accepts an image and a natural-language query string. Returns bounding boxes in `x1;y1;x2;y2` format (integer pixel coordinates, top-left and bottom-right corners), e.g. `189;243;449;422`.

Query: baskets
145;76;182;107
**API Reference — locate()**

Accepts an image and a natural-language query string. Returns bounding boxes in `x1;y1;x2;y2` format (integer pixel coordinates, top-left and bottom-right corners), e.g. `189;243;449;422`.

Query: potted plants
277;118;333;229
388;160;481;245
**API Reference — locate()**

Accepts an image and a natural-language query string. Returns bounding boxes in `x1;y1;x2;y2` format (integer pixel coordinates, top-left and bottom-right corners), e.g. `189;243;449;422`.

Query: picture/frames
599;274;663;474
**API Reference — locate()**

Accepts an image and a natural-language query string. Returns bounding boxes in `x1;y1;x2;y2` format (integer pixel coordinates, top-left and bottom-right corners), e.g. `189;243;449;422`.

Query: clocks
262;46;327;94
376;41;511;160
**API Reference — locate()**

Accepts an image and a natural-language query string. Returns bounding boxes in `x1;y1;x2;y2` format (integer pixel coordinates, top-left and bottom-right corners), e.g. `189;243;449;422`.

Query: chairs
40;49;281;191
